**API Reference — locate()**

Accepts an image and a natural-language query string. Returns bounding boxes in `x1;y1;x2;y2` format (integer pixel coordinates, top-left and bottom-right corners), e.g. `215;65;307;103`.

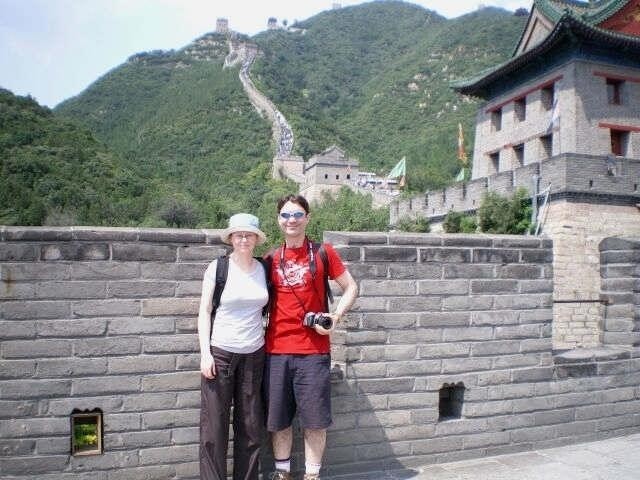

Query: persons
197;211;271;480
264;195;359;479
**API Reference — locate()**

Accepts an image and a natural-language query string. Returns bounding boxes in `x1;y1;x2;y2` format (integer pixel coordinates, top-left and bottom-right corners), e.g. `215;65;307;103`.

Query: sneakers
268;468;293;480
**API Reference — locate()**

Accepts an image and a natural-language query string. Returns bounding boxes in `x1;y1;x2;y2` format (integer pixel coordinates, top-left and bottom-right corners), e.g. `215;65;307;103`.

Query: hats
221;213;266;247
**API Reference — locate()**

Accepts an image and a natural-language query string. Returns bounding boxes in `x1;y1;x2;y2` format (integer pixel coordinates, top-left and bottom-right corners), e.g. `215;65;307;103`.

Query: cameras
302;312;333;329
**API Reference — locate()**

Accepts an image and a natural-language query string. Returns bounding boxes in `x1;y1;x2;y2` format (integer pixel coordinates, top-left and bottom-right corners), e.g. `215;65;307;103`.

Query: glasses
234;234;255;242
280;211;304;219
